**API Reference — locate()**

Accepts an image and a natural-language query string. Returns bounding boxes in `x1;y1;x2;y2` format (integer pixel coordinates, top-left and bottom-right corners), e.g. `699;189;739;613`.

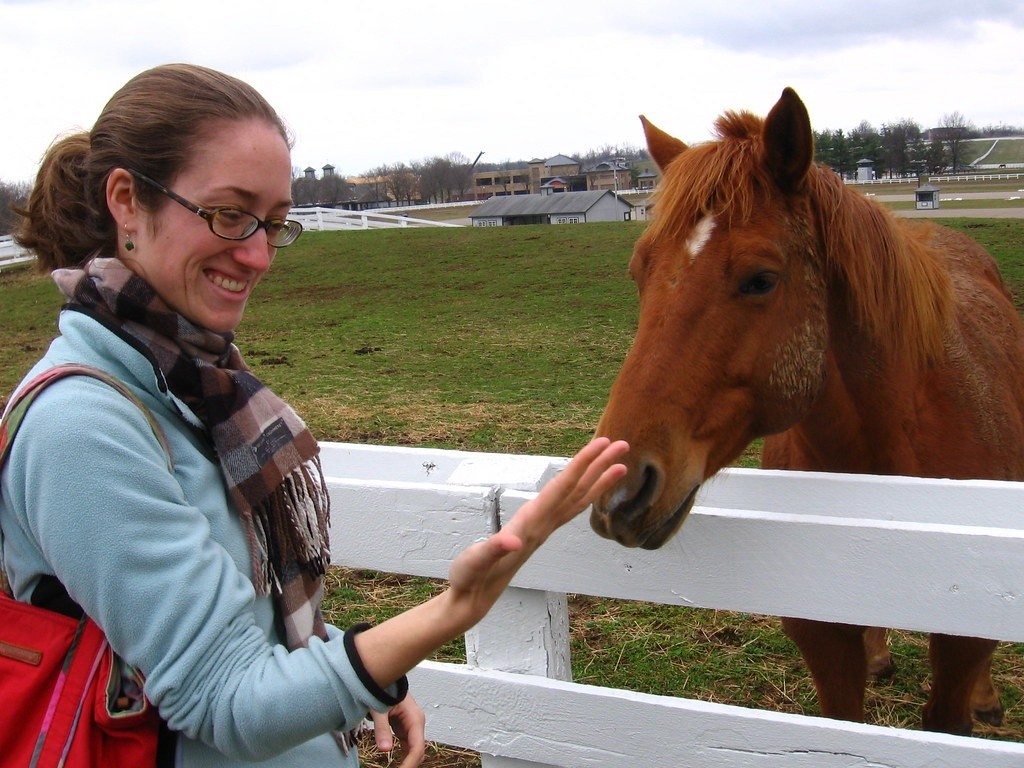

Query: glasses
126;168;303;248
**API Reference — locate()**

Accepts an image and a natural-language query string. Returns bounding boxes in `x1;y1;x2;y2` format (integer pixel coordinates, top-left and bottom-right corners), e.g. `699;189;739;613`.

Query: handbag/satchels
0;362;179;768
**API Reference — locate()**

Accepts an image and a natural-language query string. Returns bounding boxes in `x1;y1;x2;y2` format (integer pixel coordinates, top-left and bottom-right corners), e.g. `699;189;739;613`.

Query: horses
589;85;1024;737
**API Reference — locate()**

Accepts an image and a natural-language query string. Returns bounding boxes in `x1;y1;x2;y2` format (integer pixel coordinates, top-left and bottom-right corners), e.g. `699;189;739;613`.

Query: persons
0;63;628;767
